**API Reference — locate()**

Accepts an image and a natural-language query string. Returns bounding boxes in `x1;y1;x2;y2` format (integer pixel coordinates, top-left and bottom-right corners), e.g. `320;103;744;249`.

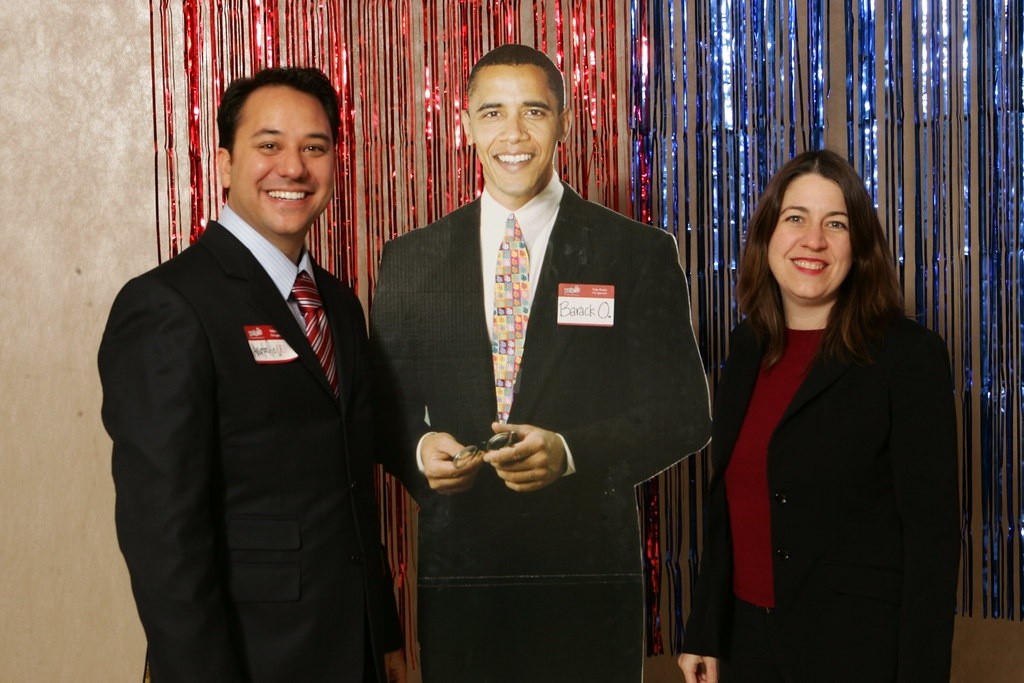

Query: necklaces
675;150;961;682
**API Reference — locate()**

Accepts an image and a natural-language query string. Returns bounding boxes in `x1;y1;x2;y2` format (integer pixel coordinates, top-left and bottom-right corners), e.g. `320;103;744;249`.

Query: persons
96;67;400;683
374;43;716;683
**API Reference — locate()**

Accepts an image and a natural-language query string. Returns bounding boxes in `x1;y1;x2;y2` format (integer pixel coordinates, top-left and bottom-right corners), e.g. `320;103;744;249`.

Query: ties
488;213;531;426
288;270;340;405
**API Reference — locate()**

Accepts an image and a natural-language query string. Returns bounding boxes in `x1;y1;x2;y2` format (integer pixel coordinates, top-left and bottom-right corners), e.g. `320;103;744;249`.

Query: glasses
453;430;519;469
